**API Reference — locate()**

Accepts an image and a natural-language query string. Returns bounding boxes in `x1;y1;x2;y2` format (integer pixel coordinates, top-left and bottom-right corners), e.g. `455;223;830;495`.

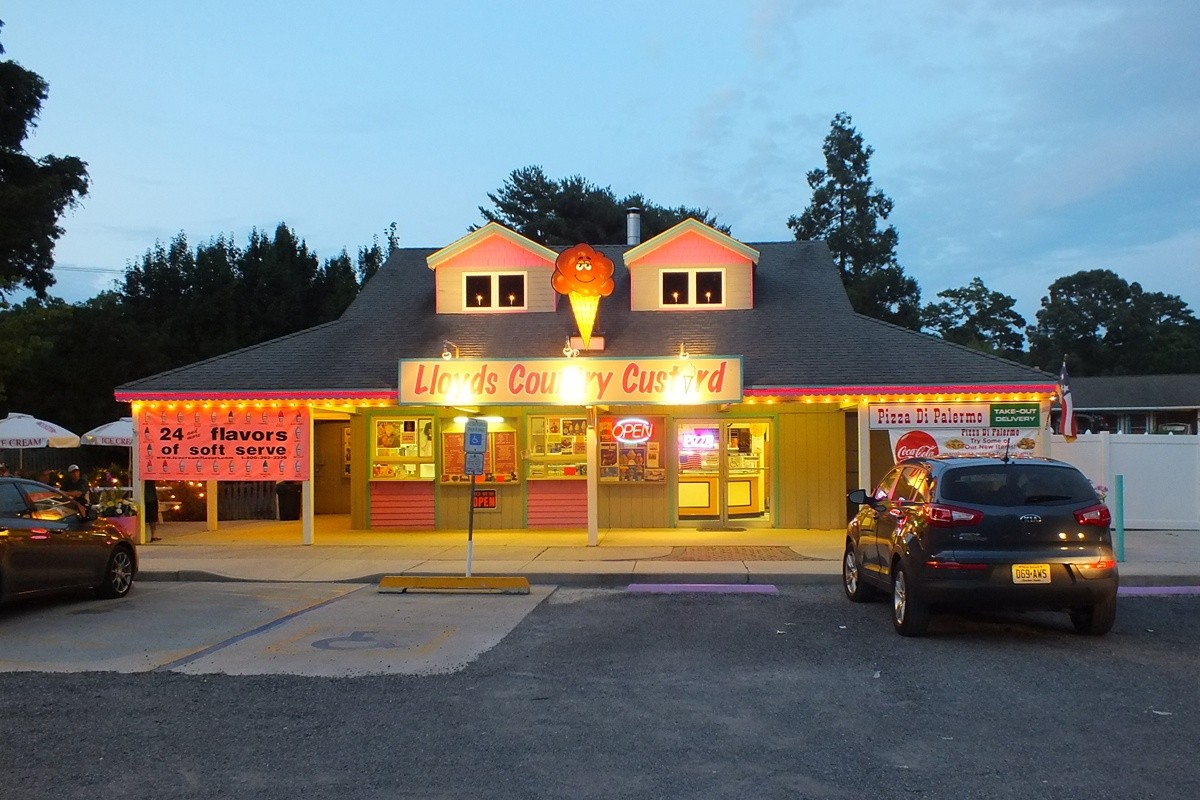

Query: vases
107;513;137;540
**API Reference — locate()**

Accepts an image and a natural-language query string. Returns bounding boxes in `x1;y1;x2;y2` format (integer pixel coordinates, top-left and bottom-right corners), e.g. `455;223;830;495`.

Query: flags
1056;361;1077;443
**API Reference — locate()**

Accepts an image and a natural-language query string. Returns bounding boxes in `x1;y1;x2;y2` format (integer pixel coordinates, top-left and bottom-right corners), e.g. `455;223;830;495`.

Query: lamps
679;341;691;360
442;340;460;361
562;336;580;358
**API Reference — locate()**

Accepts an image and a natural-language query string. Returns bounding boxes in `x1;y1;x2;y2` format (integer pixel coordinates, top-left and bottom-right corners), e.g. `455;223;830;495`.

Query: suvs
841;452;1119;639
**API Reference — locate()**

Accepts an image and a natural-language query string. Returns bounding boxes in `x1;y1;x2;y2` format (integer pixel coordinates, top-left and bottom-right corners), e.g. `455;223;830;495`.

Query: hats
67;465;79;472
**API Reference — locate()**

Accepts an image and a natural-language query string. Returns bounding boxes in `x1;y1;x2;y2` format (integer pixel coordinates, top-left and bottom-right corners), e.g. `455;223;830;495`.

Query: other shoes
151;537;162;542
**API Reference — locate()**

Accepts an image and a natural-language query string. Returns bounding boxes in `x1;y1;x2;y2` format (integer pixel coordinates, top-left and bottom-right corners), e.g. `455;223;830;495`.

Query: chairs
941;482;987;505
1020;478;1068;504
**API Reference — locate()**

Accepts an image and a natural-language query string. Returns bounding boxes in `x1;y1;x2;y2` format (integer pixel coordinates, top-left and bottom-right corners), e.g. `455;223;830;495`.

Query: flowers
95;498;138;519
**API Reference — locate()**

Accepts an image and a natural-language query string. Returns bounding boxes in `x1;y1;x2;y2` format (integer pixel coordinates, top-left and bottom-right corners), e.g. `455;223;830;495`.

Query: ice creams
619;450;643;465
145;410;301;473
569;421;586;435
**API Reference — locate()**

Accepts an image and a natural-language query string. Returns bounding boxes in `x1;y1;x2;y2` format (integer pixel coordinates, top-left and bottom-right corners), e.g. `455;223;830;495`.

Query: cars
0;476;138;612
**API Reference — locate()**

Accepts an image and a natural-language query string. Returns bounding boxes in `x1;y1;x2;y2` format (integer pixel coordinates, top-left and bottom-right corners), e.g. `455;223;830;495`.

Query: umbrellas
80;417;133;497
0;413;81;468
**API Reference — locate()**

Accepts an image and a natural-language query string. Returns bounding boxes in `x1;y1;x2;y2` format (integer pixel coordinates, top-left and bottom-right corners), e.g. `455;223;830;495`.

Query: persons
60;464;89;504
145;480;163;542
